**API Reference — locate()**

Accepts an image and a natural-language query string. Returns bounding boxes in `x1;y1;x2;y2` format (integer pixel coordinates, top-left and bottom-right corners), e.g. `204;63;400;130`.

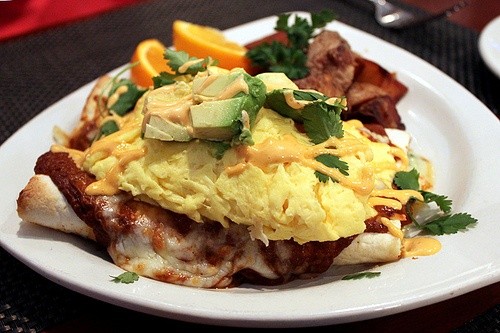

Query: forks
365;0;469;28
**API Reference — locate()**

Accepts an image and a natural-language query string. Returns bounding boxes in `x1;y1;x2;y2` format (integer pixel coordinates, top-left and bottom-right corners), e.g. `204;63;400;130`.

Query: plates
0;10;500;328
477;13;500;79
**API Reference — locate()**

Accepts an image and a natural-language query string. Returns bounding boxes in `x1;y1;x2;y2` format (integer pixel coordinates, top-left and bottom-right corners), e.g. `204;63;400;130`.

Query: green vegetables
96;6;478;235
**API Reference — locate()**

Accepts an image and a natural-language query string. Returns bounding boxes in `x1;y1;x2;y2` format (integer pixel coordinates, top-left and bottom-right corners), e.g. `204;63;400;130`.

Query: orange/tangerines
129;37;175;88
172;19;263;74
